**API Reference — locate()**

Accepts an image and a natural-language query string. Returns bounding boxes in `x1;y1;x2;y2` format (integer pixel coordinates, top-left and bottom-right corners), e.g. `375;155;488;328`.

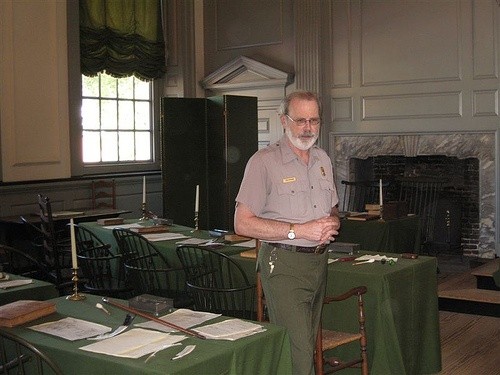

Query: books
0;300;56;329
130;225;170;233
97;218;123;226
346;214;380;221
225;234;250;241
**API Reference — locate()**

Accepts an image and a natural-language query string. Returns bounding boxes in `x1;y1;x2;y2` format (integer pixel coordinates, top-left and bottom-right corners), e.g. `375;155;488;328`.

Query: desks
335;214;423;254
1;208;133;282
87;220;443;374
0;272;59;306
0;291;296;375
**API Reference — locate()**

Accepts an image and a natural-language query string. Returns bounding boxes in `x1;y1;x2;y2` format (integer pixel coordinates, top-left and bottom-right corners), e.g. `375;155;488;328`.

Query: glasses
286;113;321;127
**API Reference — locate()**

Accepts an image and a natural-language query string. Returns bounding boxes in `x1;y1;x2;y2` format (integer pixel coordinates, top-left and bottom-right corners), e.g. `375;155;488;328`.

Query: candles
195;184;199;211
70;218;77;268
143;176;146;204
380;178;382;205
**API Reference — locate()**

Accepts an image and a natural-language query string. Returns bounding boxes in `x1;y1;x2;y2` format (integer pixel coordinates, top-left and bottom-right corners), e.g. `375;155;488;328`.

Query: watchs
288;224;295;239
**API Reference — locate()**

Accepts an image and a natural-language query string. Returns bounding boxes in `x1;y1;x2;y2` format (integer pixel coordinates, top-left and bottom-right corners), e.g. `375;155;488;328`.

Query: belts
269;242;329;255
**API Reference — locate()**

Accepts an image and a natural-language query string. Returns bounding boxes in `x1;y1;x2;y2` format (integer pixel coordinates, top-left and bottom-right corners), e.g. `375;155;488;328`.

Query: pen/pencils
84;314;133;340
170;345;196;360
145;343;182;363
96;303;111;315
352;259;374;266
198;243;229;247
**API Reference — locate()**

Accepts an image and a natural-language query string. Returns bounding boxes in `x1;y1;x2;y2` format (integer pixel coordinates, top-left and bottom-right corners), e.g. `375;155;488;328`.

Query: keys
269;261;275;273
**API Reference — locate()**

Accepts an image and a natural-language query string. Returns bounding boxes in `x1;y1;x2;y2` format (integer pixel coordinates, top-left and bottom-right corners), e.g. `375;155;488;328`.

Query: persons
234;90;340;375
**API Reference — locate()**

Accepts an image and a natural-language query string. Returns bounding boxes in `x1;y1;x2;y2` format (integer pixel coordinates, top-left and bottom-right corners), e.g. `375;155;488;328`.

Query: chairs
0;178;446;375
